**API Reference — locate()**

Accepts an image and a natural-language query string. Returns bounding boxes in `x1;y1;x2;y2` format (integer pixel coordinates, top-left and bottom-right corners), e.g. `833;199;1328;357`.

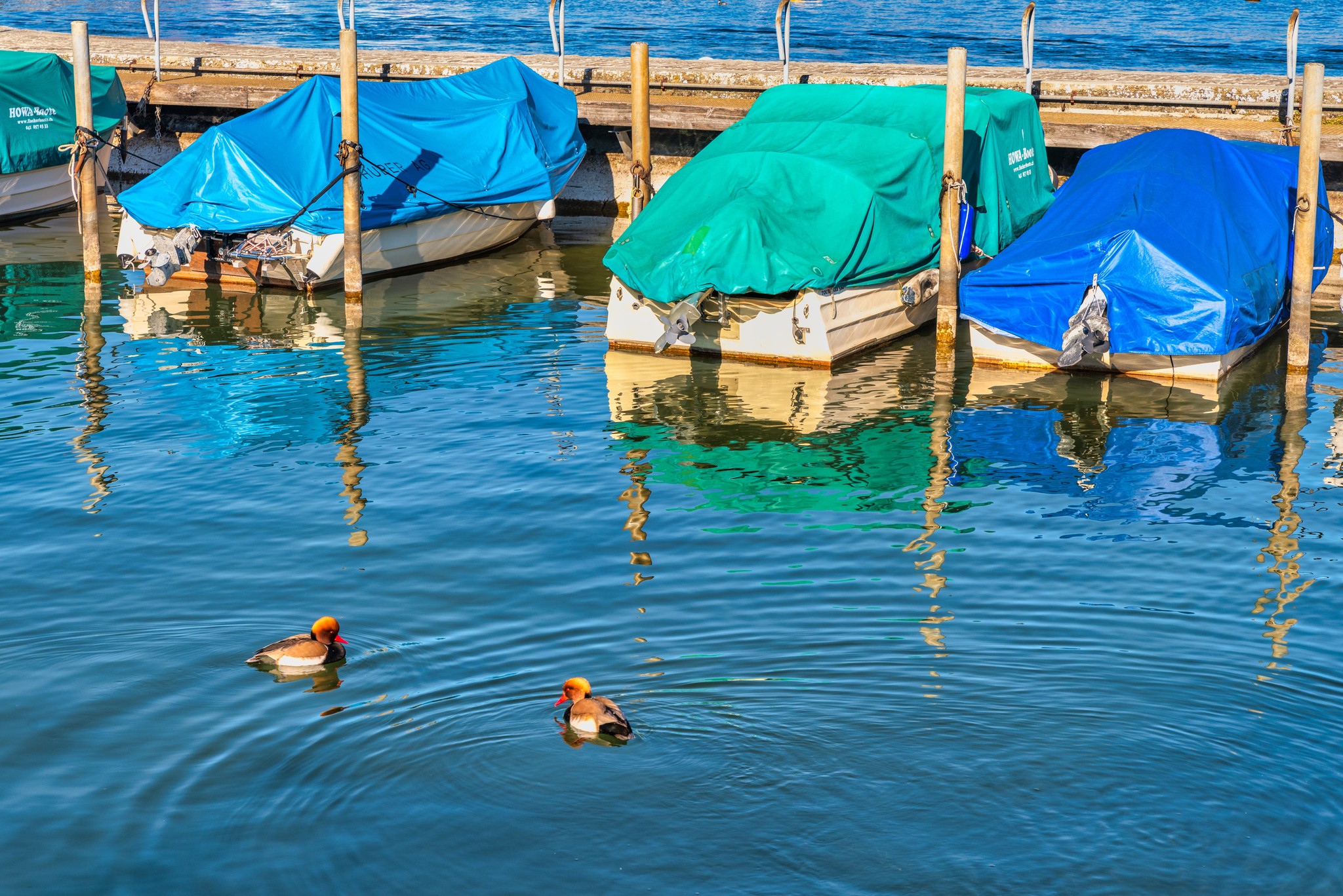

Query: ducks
553;676;636;742
242;615;350;667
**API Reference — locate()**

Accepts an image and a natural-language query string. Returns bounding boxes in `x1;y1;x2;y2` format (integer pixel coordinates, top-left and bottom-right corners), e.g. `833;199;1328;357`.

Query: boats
604;83;1058;368
0;49;128;224
959;125;1334;383
114;56;587;293
